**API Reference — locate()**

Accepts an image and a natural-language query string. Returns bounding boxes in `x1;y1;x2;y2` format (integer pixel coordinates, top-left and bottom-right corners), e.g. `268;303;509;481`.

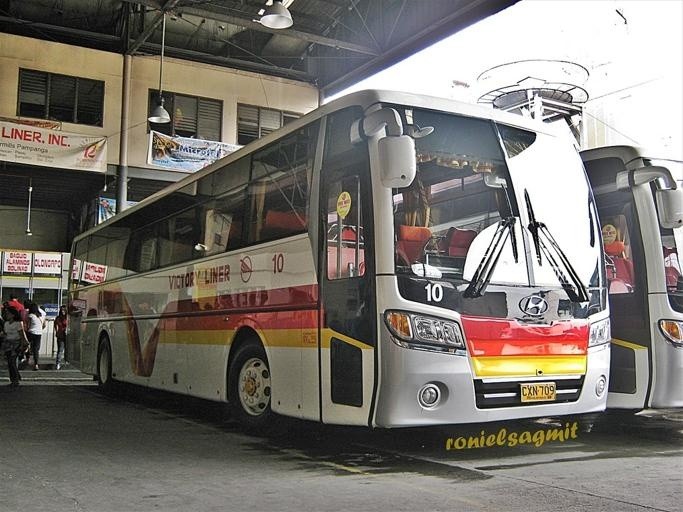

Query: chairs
230;211;477;281
608;255;679;293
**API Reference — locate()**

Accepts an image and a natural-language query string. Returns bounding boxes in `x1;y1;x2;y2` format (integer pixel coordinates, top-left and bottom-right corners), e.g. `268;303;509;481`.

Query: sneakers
65;361;69;365
56;363;61;370
24;360;31;365
33;366;39;370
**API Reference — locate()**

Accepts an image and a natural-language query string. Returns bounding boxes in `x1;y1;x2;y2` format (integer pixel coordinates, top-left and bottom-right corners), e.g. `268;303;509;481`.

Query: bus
63;89;614;433
579;143;683;421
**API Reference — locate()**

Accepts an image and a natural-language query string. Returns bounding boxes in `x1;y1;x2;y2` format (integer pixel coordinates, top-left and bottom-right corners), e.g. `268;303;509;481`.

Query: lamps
259;0;294;29
147;11;171;124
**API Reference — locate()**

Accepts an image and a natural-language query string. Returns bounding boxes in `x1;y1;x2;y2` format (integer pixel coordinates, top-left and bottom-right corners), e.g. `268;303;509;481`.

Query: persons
54;305;70;370
0;293;46;387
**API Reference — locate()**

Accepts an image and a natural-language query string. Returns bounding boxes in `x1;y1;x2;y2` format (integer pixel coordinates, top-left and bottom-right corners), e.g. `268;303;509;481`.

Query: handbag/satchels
15;336;30;356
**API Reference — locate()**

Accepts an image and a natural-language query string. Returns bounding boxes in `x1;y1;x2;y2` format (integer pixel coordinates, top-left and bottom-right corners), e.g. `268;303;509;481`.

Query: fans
8;186;46;239
